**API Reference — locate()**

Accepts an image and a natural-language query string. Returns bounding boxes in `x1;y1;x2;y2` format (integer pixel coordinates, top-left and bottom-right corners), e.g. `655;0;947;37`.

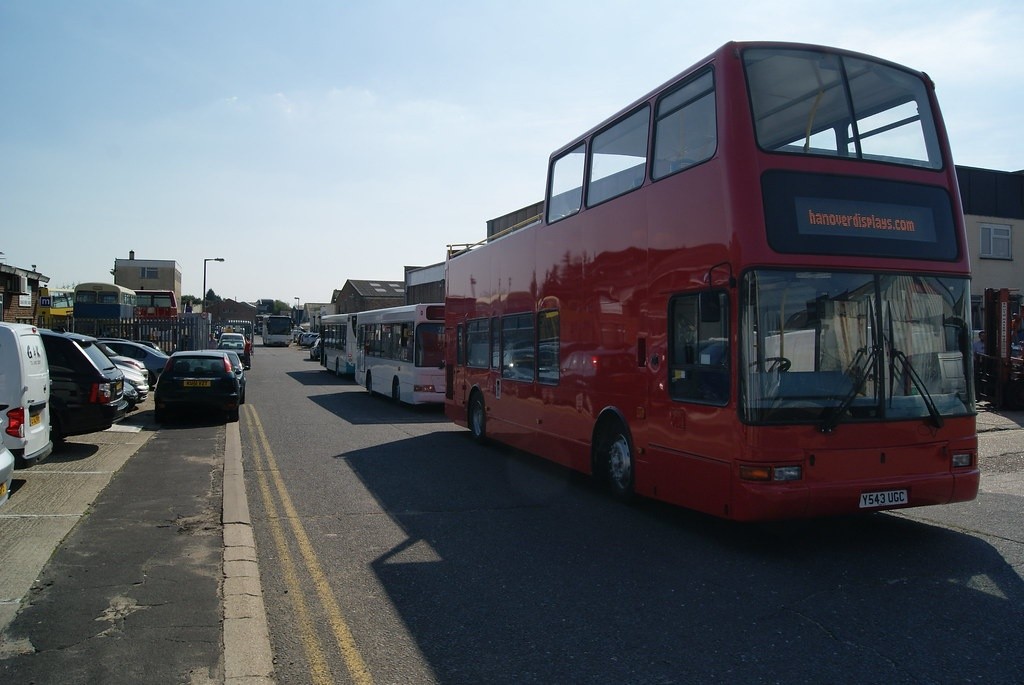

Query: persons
243;336;252;370
1011;313;1021;342
974;331;985;354
207;334;217;349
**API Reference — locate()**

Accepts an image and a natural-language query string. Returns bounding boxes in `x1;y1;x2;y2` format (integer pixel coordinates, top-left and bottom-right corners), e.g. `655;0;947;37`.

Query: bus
263;315;293;347
356;303;445;407
39;287;75;331
320;313;358;378
133;289;179;332
256;315;263;335
74;282;137;339
444;40;980;522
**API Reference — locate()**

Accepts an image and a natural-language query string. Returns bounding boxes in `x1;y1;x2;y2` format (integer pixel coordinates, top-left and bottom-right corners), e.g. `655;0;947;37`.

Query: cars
197;350;246;403
217;339;245;365
154;350;241;425
219;332;246;345
294;326;320;361
0;322;171;507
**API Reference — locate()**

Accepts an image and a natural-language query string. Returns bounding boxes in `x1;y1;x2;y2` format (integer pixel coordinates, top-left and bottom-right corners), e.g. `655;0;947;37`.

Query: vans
226;321;253;354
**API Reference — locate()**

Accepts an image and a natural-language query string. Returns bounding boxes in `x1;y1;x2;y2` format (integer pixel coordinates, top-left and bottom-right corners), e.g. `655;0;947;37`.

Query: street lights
295;297;300;342
203;258;225;313
